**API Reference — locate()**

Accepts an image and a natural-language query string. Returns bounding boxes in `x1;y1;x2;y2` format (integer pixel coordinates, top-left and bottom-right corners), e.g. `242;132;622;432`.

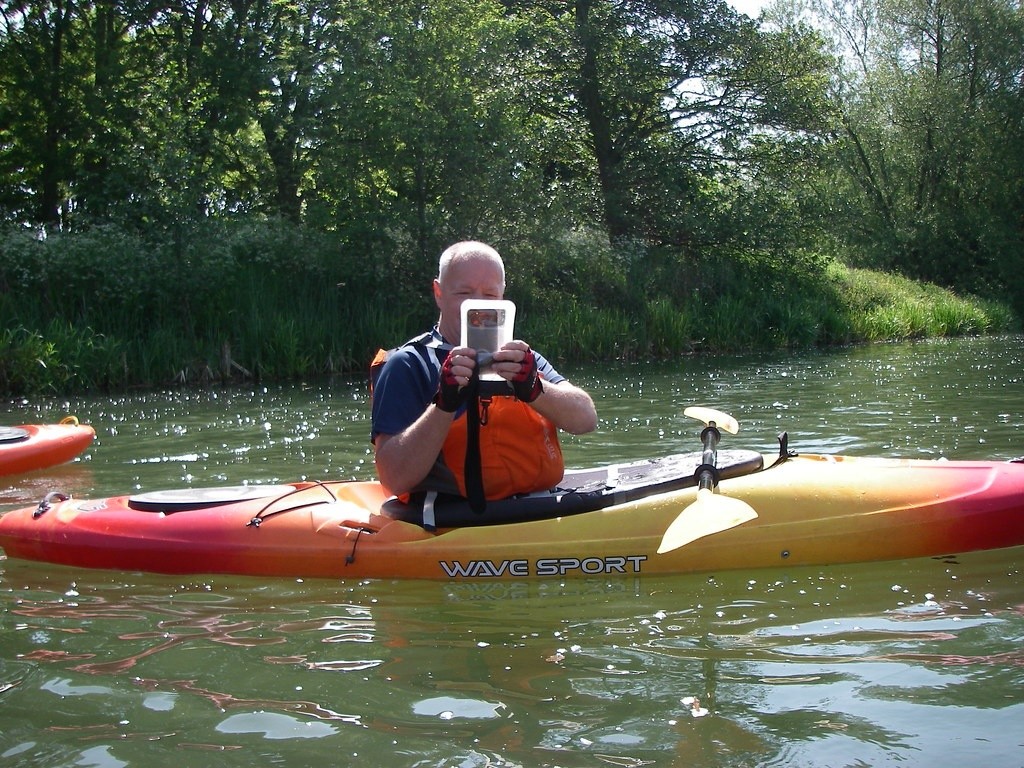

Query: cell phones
468;309;498;375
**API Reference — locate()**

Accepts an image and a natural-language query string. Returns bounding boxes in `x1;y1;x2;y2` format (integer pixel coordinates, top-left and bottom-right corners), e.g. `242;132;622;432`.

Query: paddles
656;405;760;556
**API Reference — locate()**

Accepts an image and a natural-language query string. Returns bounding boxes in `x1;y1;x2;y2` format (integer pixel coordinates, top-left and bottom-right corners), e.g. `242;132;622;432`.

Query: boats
1;414;95;477
0;431;1024;582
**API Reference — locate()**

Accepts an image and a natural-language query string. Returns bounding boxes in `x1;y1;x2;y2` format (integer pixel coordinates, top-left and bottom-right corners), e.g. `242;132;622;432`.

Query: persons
371;241;598;525
